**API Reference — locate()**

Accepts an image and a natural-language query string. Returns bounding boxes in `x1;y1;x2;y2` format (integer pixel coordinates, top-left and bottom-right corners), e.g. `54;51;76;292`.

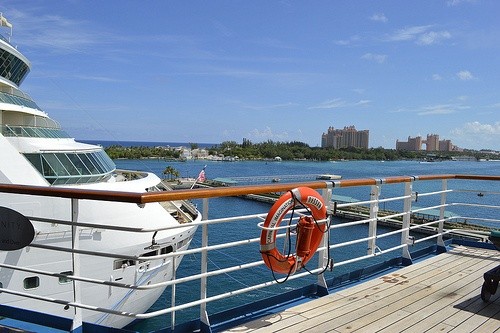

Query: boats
0;9;215;333
158;170;199;189
316;173;343;180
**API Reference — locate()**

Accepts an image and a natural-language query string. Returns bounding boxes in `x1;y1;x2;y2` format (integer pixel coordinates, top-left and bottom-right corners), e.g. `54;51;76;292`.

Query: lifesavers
258;186;327;275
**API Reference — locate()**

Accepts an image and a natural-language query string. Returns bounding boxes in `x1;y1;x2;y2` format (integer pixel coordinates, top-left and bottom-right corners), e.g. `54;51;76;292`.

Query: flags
198;170;206;184
